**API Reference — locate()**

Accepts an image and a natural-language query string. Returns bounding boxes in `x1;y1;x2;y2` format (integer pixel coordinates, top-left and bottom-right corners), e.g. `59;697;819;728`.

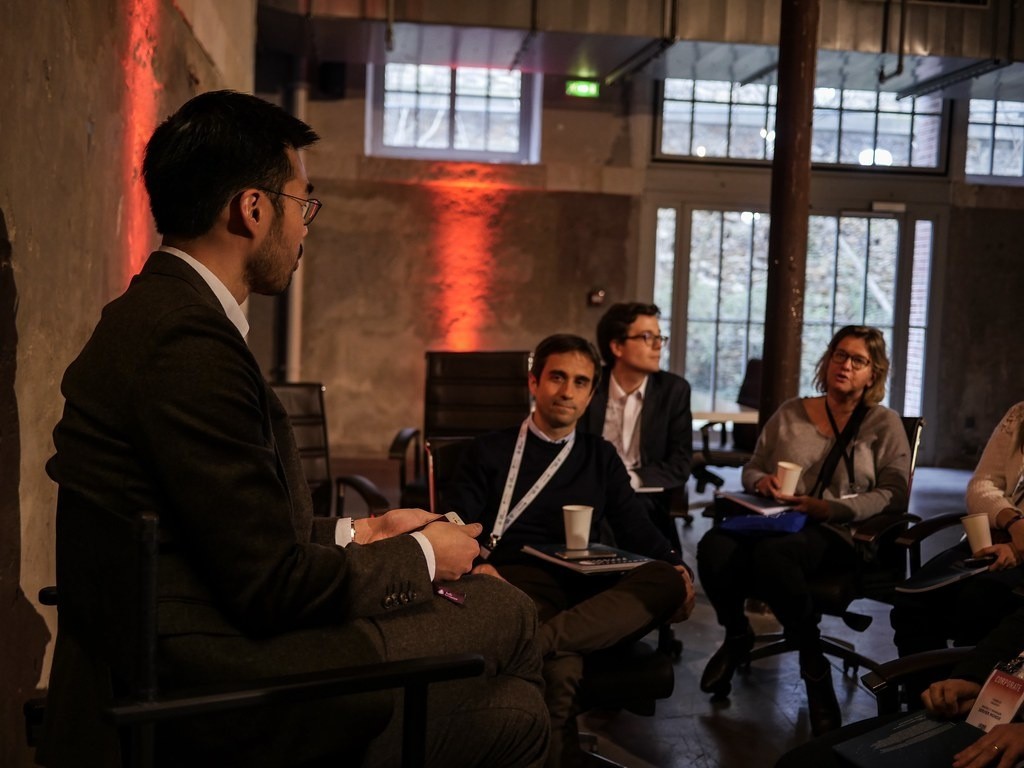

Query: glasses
258;187;322;227
625;330;669;349
831;346;873;370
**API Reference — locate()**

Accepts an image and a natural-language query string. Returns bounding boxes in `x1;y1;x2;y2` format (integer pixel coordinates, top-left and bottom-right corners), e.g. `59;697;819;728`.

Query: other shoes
799;656;843;737
698;618;757;694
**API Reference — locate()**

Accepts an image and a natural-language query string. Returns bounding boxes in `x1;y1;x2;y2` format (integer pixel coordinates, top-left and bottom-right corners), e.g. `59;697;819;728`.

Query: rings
993;744;999;753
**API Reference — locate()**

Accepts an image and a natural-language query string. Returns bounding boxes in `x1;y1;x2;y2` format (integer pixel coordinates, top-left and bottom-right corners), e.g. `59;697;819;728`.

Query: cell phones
556;549;617;560
396;510;465;536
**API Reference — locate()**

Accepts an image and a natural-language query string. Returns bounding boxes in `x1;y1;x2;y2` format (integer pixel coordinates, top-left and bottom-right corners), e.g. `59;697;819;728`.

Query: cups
562;505;594;549
959;512;991;553
774;461;802;503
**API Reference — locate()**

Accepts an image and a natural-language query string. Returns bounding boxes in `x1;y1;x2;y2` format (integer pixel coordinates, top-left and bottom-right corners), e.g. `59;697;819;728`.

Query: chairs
690;358;763;494
22;350;693;768
716;417;1024;717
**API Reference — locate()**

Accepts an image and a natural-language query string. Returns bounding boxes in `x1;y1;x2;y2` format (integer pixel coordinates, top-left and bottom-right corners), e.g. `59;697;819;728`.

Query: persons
574;302;694;576
700;325;912;693
38;88;550;768
921;648;1024;768
964;400;1024;572
444;333;694;768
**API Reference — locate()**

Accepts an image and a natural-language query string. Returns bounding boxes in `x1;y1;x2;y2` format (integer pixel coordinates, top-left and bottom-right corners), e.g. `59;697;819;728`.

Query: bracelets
1003;515;1024;527
